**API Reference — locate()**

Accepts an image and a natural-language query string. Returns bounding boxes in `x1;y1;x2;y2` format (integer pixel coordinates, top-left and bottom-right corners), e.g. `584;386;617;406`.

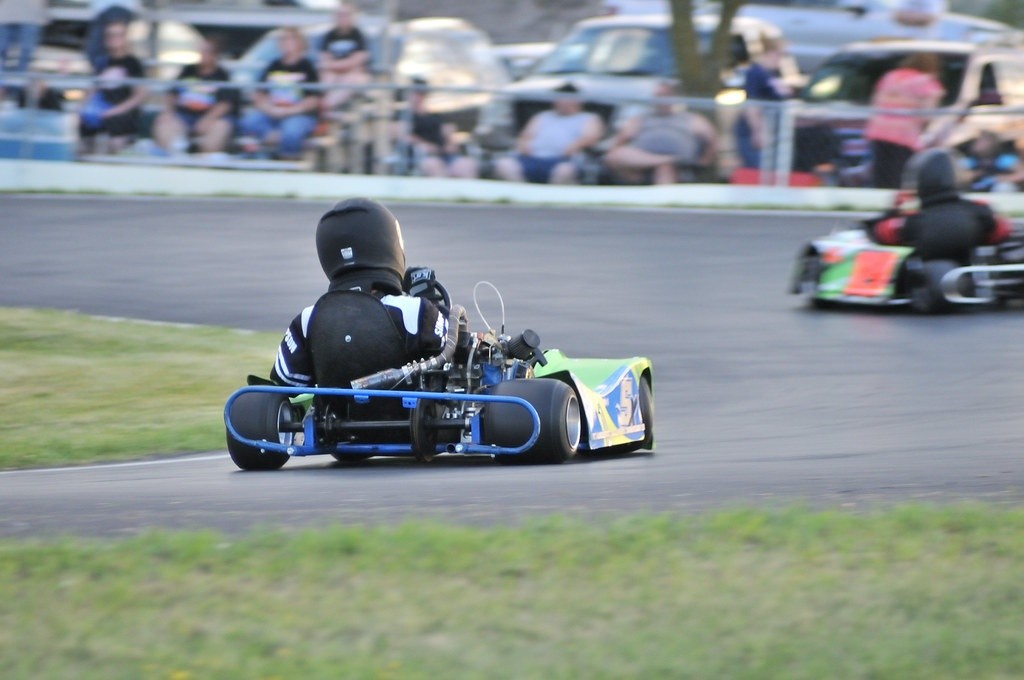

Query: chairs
912;196;980;264
303;292;414;421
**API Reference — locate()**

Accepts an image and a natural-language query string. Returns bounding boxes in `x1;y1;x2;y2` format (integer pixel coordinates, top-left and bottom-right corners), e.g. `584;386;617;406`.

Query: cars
793;42;1024;173
512;12;790;137
0;10;207;112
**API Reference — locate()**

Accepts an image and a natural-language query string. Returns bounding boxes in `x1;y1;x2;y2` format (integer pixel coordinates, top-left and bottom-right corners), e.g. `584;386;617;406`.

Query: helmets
316;197;405;282
918;154;954;200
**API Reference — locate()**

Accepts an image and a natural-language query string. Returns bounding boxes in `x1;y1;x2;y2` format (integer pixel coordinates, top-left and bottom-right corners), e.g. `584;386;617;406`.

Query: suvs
226;17;512;150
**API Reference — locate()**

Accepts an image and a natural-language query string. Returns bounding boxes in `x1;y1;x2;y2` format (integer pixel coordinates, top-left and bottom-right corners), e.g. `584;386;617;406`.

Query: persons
270;198;484;443
863;148;1010;258
0;0;1024;200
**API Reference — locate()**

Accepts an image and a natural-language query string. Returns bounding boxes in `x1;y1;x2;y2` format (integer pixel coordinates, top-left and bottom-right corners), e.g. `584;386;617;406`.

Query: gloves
403;266;436;299
